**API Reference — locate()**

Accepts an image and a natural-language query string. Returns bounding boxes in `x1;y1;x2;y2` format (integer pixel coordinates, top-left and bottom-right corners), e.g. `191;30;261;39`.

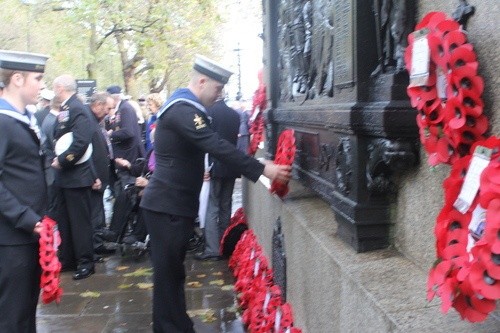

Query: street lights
233;42;243;101
53;5;97;79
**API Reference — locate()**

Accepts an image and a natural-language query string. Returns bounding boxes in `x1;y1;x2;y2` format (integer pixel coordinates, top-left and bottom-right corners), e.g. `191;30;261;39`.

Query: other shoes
194;251;219;260
186;237;200;251
97;230;118;242
122;233;137;245
93;246;107;258
106;194;114;201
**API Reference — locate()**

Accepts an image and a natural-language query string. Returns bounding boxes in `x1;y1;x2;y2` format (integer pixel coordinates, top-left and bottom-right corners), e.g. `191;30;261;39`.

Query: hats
0;50;50;74
193;53;235;84
40;89;55;100
107;86;122;95
54;132;93;165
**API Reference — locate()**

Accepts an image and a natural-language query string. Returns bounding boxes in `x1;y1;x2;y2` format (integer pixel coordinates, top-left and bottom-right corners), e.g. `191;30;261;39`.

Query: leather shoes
60;263;74;272
73;268;93;280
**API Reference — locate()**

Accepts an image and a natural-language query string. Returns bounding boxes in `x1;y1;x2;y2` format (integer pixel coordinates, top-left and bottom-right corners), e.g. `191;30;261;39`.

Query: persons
0;49;293;333
139;53;291;333
